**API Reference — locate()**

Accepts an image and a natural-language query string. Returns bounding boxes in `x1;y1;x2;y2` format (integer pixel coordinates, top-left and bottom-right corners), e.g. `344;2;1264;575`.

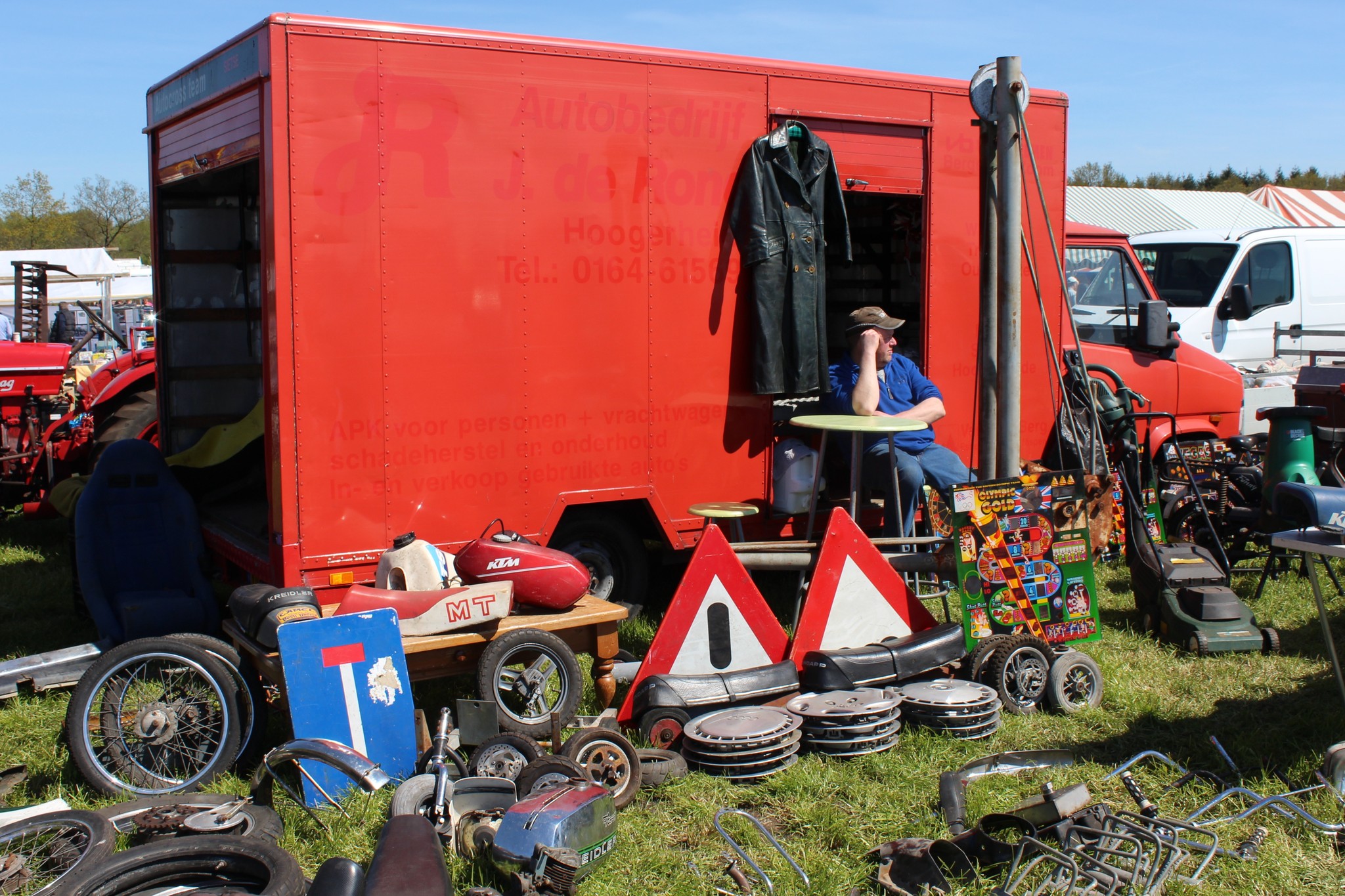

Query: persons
140;298;153;307
49;301;76;345
99;299;123;350
1067;276;1079;307
823;307;978;553
0;306;12;341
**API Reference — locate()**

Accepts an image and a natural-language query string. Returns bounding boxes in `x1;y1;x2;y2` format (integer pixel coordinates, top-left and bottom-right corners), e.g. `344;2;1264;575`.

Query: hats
844;306;906;330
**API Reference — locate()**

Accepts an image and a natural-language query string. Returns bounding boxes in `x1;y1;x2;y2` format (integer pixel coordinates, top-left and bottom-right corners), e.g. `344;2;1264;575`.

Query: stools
901;484;953;626
688;502;760;581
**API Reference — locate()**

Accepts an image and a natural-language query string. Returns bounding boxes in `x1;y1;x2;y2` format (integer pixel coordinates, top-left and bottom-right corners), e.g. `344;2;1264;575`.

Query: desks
789;416;929;637
219;592;628;711
1271;526;1345;703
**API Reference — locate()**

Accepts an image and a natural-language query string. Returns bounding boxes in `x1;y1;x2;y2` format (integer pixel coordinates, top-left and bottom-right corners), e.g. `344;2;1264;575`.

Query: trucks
135;13;1241;682
1060;217;1345;447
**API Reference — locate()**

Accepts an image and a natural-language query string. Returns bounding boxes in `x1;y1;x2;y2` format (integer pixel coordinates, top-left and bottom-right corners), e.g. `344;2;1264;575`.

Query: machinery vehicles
1;322;162;519
